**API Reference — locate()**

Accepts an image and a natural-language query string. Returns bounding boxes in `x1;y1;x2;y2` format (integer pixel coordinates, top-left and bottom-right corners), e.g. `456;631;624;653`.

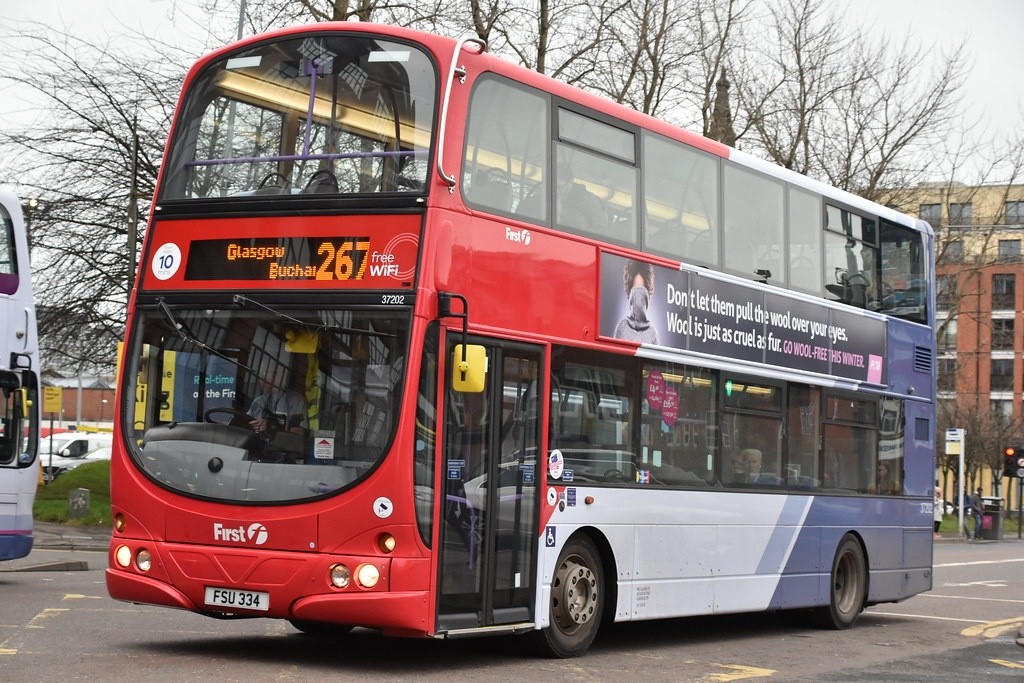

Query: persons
971;487;984;540
512;359;558;459
556;162;607;234
867;462;901;495
613;258;658;346
933;479;943;538
801;427;838;488
245;354;309;437
723;449;779;489
955;486;973;541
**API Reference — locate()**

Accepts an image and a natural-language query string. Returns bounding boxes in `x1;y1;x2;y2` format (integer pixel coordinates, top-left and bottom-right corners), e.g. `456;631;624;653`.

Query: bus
104;22;939;659
0;192;40;562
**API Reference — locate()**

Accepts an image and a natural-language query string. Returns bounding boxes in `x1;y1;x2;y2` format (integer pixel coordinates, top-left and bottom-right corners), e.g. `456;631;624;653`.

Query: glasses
258;371;291;377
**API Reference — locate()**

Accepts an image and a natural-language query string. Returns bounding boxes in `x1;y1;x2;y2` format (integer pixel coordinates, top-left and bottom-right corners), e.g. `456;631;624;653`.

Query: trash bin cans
979;496;1006;539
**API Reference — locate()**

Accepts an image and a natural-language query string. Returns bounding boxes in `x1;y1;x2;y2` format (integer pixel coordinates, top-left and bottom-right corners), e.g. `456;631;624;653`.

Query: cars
935;487;1005;534
55;446;112;482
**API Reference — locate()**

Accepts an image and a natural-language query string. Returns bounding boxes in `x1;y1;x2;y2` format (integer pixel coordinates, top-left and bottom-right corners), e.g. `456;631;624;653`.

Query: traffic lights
1003;446;1018;478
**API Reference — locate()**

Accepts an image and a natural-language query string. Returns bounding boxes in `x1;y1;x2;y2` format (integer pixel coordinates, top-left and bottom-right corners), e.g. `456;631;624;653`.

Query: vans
40;431;111;486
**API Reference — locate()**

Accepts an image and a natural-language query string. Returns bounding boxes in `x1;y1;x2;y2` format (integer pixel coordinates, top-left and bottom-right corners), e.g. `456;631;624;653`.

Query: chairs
251;168;900;321
